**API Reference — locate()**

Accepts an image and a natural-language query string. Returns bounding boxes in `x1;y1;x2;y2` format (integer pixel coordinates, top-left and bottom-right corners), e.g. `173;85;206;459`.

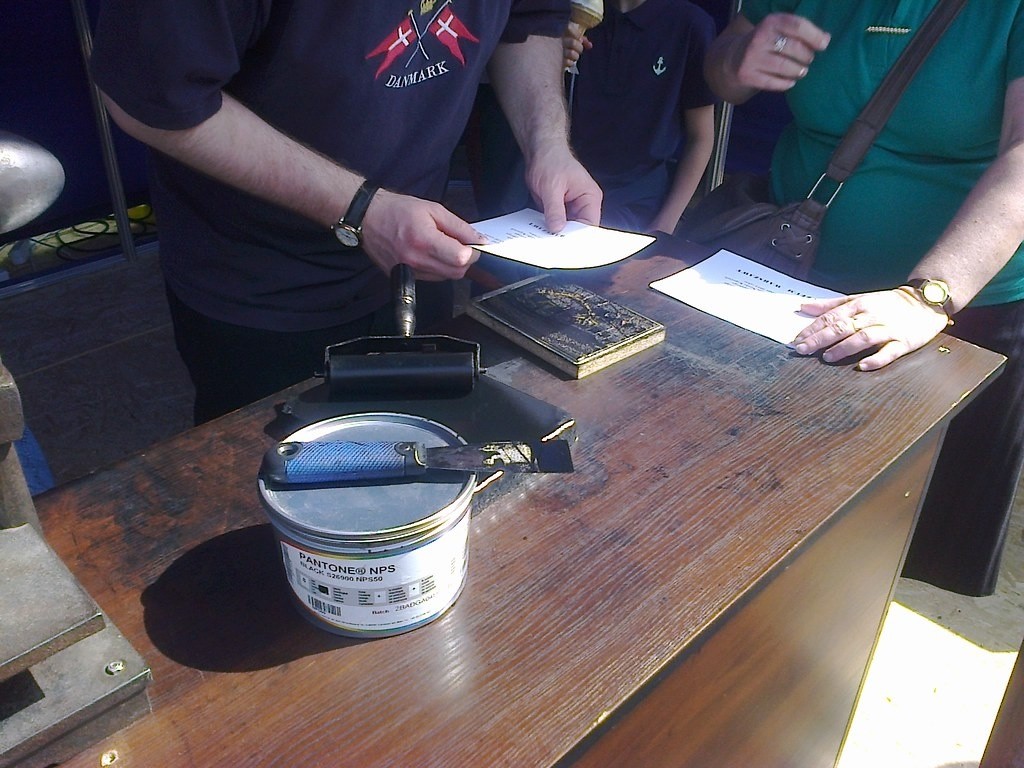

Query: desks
0;236;1011;768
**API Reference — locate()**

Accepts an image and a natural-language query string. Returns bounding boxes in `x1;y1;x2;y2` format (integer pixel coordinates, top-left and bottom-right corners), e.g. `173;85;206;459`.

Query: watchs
903;278;955;321
331;180;381;248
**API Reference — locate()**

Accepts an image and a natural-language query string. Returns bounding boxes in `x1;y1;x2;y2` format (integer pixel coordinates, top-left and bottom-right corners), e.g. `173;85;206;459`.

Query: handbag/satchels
677;179;830;285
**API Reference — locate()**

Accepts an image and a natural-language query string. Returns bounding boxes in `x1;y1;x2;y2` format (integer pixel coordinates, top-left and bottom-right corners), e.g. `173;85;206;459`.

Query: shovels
264;439;574;486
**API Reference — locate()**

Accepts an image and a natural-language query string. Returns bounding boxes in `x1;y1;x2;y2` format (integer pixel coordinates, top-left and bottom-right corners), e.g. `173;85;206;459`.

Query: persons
486;0;717;236
89;0;603;426
704;1;1024;598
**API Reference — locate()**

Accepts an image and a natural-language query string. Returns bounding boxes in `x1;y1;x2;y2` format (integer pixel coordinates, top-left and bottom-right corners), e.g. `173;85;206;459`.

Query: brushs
312;263;490;392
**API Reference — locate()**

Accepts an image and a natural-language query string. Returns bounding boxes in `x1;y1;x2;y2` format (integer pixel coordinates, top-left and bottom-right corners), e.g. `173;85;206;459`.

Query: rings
774;34;788;54
